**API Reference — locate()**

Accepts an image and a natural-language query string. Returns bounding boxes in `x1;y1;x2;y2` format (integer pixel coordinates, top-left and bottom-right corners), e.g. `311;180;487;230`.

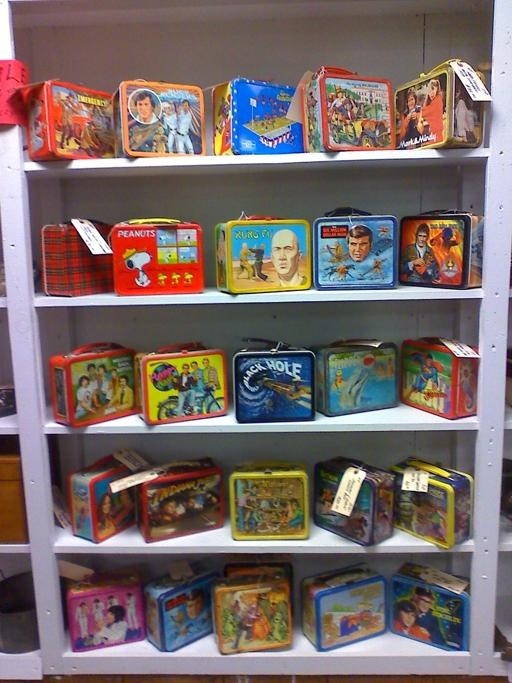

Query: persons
187;589;204;619
454;85;476;142
403;224;438;283
330;85;358;120
175;358;219;415
75;592;139;646
237;483;304;535
132;91;198;157
400;77;443;147
270;229;307;287
218;230;226;283
393;584;446;646
404;353;438;401
239;243;254;278
97;493;117;534
347;225;372;262
73;363;134;420
251;244;268;280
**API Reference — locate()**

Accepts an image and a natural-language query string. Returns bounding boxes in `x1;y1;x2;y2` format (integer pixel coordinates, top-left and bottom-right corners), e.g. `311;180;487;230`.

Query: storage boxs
0;453;29;544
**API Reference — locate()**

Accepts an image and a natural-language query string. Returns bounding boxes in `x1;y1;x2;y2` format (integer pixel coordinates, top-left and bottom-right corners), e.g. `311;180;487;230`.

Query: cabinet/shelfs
0;0;512;681
